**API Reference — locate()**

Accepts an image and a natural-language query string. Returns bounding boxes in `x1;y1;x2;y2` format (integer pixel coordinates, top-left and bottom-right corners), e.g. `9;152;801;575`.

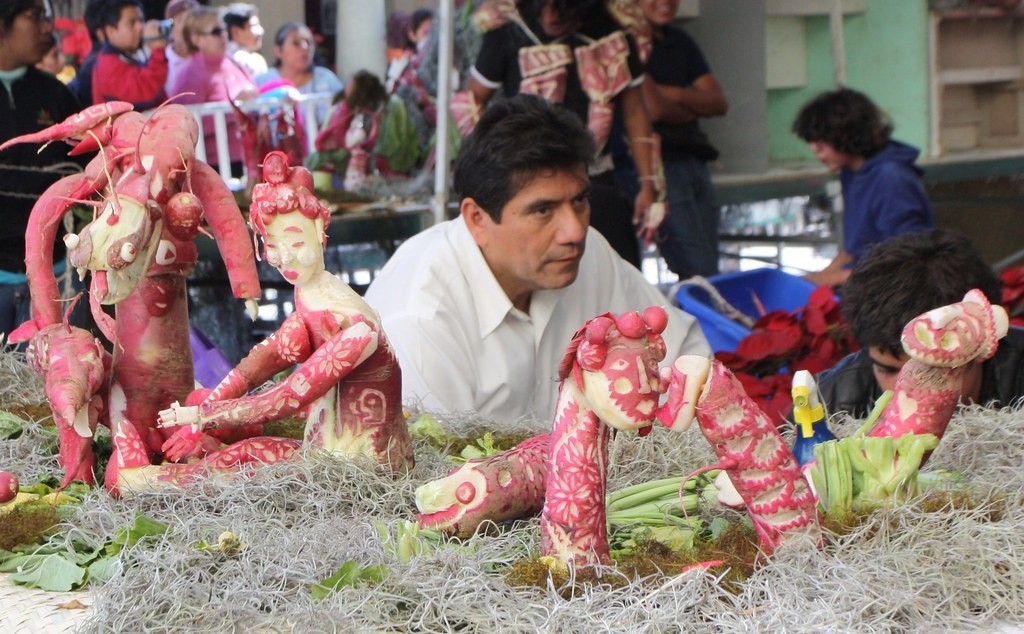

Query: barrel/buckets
677;266;846;400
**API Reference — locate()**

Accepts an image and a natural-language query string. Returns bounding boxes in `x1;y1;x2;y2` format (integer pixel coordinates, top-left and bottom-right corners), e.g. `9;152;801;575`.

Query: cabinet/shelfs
928;7;1024;159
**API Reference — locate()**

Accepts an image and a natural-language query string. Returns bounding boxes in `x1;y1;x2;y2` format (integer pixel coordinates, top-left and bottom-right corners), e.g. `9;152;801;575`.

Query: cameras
156;16;177;45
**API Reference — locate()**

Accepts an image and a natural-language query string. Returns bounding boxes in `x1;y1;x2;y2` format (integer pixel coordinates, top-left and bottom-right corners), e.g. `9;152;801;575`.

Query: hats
165;0;200;19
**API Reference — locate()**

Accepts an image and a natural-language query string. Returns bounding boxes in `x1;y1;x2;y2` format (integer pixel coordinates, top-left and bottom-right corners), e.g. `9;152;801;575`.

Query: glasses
193;26;228;37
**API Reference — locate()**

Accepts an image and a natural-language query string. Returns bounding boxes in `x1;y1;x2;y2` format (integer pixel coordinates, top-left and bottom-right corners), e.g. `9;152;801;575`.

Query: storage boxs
679;265;841;465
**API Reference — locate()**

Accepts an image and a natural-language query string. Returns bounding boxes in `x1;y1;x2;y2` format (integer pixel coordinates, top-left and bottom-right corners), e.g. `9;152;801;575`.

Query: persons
448;0;666;278
867;290;1010;451
810;229;1024;416
793;90;935;293
158;153;415;475
388;8;461;93
362;94;712;432
0;0;388;355
536;307;668;573
612;0;729;282
